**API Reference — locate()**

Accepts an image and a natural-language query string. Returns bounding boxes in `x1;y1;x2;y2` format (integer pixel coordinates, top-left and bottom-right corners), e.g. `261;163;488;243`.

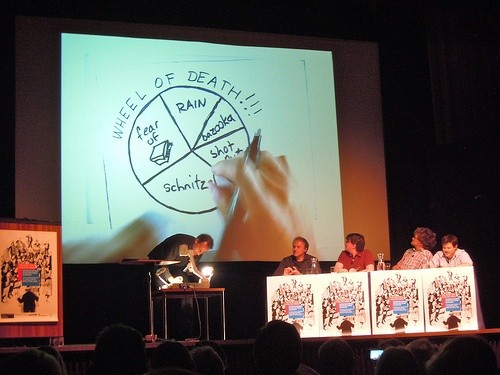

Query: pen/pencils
356;266;361;270
217;127;262;262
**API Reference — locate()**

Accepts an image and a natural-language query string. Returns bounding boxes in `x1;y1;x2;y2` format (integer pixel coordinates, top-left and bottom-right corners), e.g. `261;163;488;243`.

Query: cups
385;262;391;270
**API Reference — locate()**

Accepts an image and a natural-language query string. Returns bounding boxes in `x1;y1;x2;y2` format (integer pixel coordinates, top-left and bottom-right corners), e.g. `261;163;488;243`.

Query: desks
152;288;226;340
266;265;484;338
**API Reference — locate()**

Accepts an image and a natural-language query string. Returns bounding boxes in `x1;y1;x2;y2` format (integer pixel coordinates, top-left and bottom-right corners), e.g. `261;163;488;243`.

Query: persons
429;235;473;268
147;233;215;340
391;227;437;270
0;321;500;375
209;149;319;261
274;236;322;277
333;233;375;272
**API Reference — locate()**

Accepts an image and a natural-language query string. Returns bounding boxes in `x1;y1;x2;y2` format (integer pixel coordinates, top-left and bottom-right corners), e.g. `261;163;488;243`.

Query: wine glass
311;258;318;274
377;253;385;269
